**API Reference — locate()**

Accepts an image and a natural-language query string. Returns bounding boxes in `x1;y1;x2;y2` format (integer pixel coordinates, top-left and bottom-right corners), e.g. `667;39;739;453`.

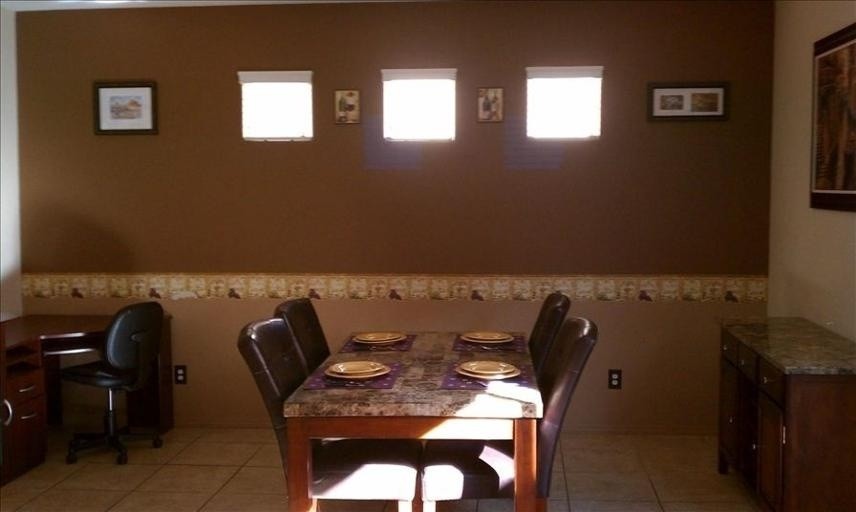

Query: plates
351;332;407;346
460;330;515;345
325;360;390;380
457;360;521;382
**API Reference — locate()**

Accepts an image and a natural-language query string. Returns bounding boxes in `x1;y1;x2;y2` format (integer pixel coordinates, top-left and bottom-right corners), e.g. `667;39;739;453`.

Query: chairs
58;302;166;468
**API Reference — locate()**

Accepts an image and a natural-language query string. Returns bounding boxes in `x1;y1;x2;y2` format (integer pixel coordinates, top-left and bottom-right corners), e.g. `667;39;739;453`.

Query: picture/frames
809;22;856;214
646;81;729;121
92;78;159;136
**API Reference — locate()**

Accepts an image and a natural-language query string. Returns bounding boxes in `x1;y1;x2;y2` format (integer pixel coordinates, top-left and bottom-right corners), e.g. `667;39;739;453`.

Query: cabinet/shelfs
716;312;856;511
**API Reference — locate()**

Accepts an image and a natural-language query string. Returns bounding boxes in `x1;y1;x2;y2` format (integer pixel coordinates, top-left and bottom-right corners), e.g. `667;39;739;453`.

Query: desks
0;311;174;489
